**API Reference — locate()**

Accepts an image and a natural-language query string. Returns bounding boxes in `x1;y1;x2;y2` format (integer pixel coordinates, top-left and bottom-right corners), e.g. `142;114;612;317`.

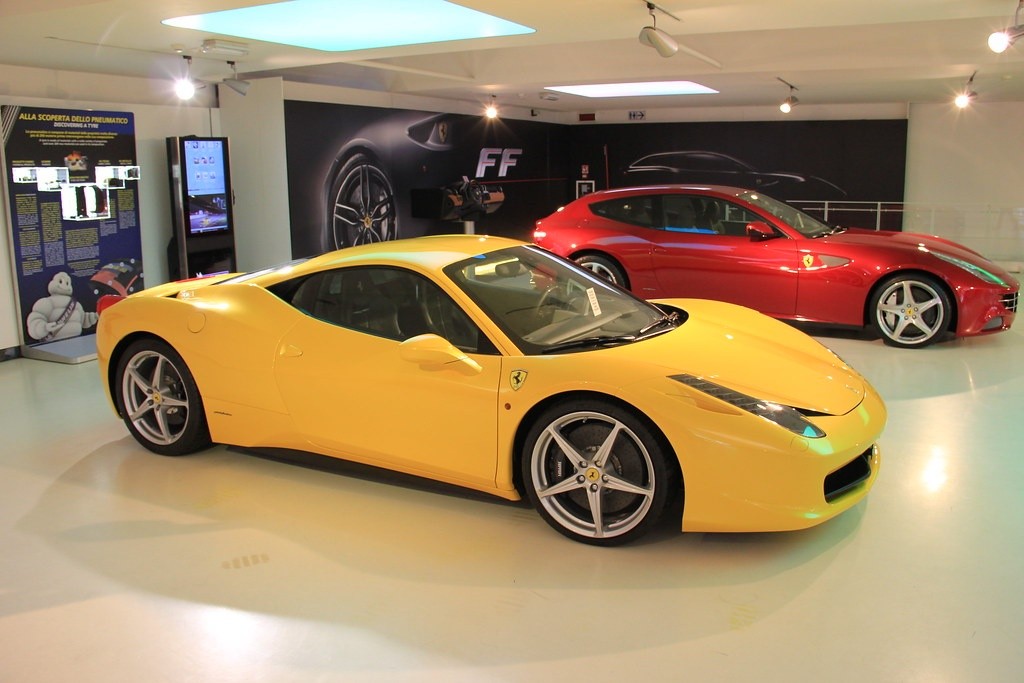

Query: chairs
318;270;481;352
672;199;727;238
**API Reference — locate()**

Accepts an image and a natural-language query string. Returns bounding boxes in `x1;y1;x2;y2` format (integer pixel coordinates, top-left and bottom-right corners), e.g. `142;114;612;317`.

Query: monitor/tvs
183;140;228;234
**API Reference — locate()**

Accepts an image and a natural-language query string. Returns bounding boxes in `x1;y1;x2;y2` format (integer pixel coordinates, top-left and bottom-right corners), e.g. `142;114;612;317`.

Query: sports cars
96;234;886;547
530;182;1019;349
318;110;531;255
622;149;847;228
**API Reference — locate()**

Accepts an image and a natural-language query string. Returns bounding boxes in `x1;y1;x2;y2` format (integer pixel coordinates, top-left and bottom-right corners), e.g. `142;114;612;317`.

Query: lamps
988;1;1024;54
641;4;679;58
957;70;977;107
781;95;800;113
179;58;205;91
200;40;250;57
224;63;251;97
